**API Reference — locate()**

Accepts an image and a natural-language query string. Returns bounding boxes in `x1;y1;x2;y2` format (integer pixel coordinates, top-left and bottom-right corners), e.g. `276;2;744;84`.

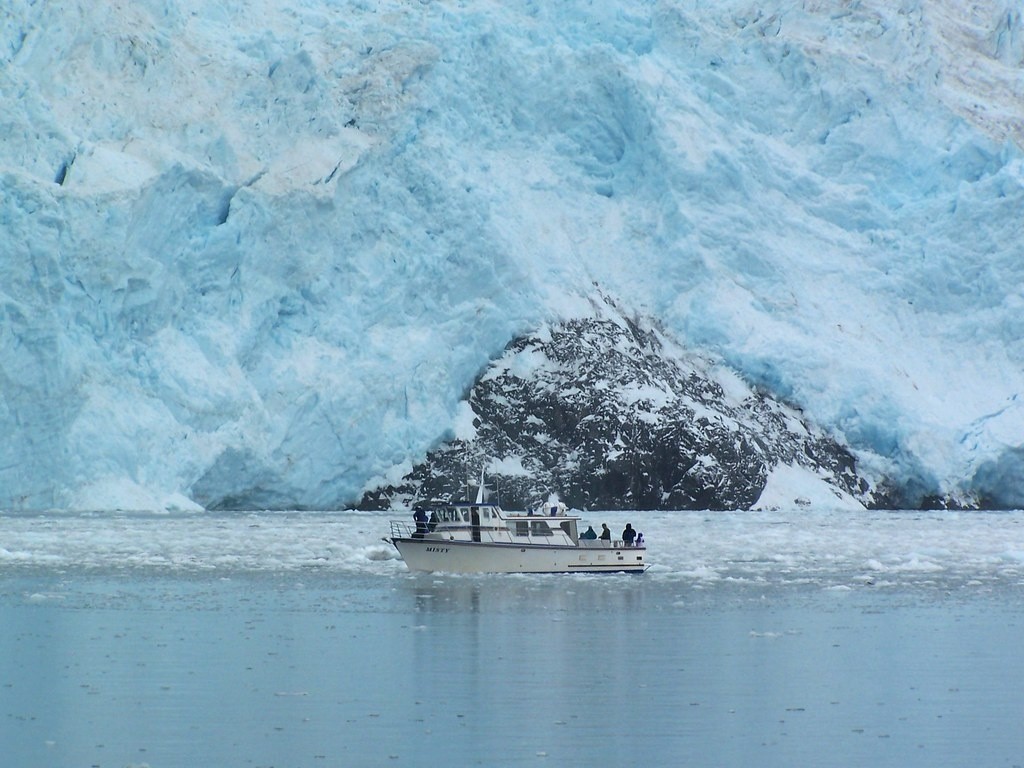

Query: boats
387;443;651;574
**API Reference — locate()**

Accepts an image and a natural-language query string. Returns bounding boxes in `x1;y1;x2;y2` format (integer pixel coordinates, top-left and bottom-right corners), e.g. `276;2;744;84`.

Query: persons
636;533;645;547
623;524;636;547
579;526;597;539
412;506;439;532
598;524;611;543
472;508;480;542
